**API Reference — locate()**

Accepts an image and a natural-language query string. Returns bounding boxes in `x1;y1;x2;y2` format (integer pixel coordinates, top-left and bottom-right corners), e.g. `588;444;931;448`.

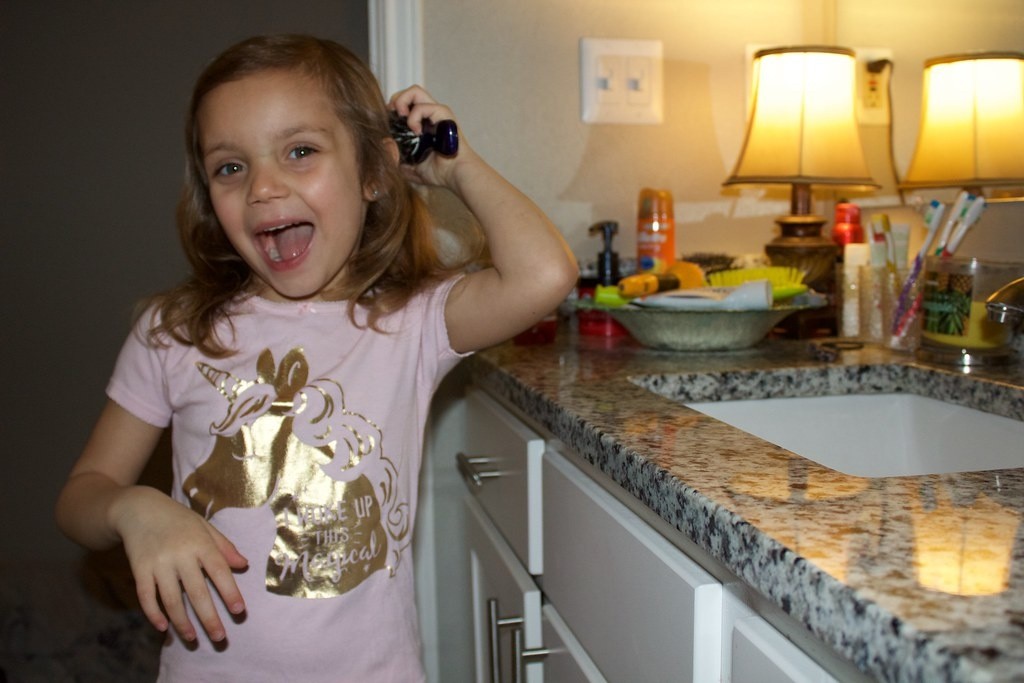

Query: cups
859;264;924;351
924;258;1013;349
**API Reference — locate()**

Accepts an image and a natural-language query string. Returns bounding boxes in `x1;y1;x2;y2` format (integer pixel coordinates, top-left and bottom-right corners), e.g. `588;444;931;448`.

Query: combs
391;109;460;163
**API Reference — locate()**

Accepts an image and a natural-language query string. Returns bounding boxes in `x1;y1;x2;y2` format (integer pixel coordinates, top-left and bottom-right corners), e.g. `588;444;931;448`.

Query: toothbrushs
870;191;988;349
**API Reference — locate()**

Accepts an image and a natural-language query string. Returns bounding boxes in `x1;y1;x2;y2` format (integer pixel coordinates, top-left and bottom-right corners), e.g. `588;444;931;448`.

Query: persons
63;38;578;681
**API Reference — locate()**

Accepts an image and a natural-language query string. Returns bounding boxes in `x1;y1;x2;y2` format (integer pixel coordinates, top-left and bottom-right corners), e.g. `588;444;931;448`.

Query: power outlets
856;62;892;129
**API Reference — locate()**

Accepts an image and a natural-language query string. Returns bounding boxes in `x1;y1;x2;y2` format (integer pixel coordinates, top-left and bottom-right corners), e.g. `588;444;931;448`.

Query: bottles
636;188;676;273
830;201;863;258
844;244;870;336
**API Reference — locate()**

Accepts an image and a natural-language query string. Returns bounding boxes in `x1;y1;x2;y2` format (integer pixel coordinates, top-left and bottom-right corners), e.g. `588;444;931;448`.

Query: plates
606;293;824;351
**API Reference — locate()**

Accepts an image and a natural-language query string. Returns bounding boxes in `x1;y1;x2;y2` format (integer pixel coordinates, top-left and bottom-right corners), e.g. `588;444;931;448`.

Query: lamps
895;52;1024;210
720;46;884;338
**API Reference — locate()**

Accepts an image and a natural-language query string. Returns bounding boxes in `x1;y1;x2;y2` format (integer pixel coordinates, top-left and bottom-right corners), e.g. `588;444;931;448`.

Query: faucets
984;275;1024;325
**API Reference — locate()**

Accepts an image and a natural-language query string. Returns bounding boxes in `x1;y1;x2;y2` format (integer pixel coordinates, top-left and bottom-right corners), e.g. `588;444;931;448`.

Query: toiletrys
830;203;911;341
512;187;676;347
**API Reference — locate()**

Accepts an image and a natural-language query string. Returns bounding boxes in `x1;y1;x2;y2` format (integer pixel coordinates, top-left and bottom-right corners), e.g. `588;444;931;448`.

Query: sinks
624;361;1024;481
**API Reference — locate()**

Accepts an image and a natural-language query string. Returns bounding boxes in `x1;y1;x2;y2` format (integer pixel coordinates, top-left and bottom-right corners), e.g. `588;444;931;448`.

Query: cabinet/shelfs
429;373;880;683
